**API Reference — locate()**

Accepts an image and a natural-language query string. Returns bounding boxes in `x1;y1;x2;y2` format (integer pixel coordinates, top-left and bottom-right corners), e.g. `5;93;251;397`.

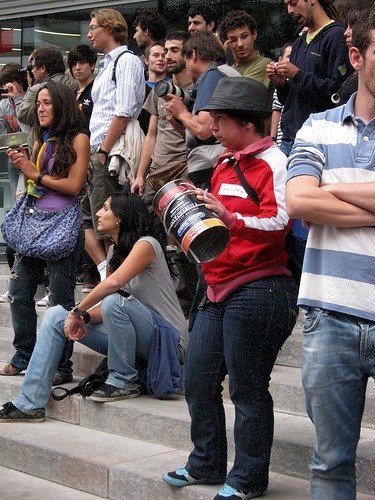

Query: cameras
0;88;8;94
155;80;196;112
18;65;35;79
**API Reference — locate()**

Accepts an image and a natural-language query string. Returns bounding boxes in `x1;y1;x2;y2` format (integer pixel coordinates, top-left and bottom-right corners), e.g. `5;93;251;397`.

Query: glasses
89;25;100;31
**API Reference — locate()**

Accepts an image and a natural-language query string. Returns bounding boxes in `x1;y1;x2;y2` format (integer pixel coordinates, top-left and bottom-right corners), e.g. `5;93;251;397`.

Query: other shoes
0;291;10;302
36;296;50;307
82;275;96;292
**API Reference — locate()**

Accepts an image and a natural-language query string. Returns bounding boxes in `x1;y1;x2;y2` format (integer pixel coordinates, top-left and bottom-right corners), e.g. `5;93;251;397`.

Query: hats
195;76;273;115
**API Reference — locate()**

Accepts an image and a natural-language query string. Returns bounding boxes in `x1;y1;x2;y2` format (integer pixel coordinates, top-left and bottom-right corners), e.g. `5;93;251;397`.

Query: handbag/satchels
0;193;82;259
51;357;108;401
146;160;187;192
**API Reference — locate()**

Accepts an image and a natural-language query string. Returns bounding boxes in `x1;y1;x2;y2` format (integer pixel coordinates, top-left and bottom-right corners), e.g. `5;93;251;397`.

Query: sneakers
214;483;265;500
0;401;46;422
90;384;140;402
164;467;224;488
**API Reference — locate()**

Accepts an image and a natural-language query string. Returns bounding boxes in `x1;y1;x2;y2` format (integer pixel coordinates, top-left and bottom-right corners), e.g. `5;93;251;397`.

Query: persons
0;80;91;386
0;0;375;319
285;8;375;500
0;191;190;423
162;77;300;500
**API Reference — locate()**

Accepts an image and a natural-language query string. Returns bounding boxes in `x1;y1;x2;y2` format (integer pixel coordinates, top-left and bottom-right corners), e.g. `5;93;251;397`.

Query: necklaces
331;73;358;103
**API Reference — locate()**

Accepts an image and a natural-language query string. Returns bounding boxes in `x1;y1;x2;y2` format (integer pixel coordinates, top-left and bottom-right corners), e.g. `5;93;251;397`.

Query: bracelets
82;312;90;325
37;171;48;185
72;306;82;318
